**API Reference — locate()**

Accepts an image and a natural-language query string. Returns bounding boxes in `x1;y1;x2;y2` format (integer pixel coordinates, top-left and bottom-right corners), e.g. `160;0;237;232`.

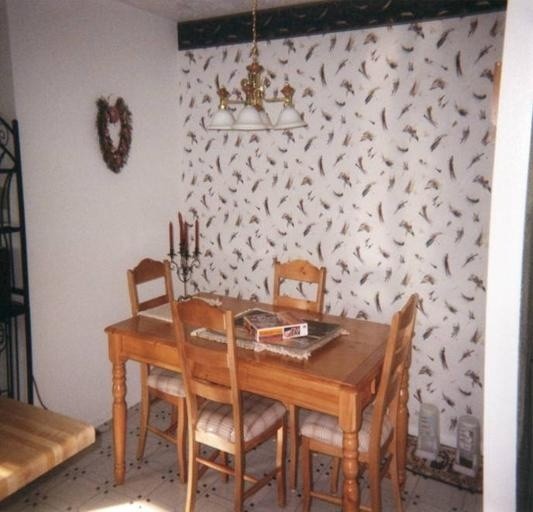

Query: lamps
203;0;307;132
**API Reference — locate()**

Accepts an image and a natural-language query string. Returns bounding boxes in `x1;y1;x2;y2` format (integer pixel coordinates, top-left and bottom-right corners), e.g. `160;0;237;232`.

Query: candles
168;209;199;251
